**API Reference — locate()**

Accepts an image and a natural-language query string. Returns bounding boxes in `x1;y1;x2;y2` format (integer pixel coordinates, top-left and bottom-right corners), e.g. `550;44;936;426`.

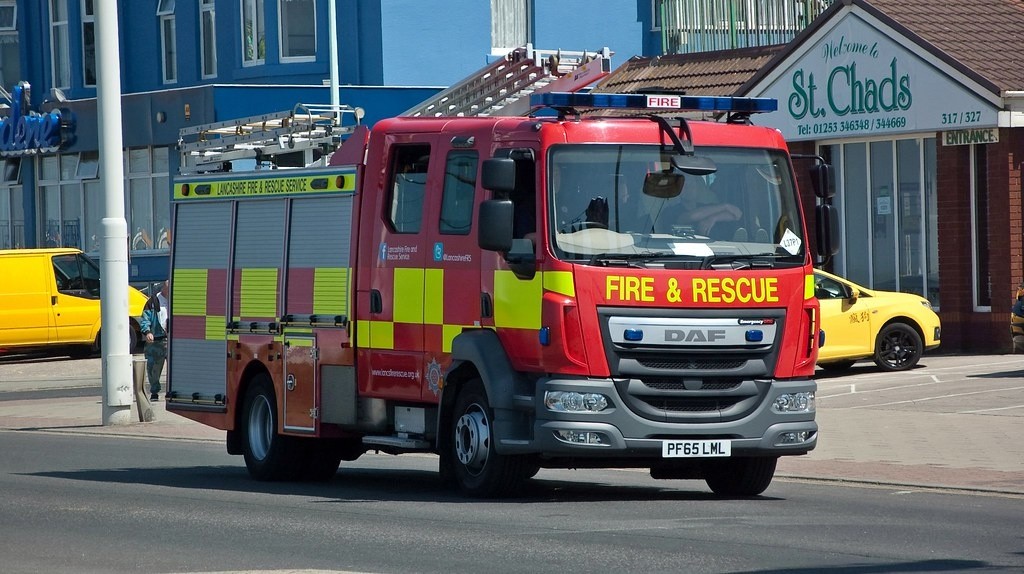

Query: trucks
0;248;149;359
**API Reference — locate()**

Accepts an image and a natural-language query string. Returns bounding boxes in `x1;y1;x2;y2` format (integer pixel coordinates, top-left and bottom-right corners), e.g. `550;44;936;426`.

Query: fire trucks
165;41;821;503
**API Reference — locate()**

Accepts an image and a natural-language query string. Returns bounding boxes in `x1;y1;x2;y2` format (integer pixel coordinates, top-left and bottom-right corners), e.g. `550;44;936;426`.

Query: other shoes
733;226;769;243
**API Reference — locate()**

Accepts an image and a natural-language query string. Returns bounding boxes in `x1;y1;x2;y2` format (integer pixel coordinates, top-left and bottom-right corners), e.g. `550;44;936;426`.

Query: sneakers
149;394;159;401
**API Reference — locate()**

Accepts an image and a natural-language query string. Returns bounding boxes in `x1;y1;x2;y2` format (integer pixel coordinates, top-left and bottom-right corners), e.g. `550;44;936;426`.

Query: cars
812;265;942;371
59;247;171;298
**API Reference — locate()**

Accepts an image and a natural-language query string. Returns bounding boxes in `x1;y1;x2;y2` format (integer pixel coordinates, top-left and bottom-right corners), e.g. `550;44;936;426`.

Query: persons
662;172;770;243
513;163;581;245
141;279;169;401
